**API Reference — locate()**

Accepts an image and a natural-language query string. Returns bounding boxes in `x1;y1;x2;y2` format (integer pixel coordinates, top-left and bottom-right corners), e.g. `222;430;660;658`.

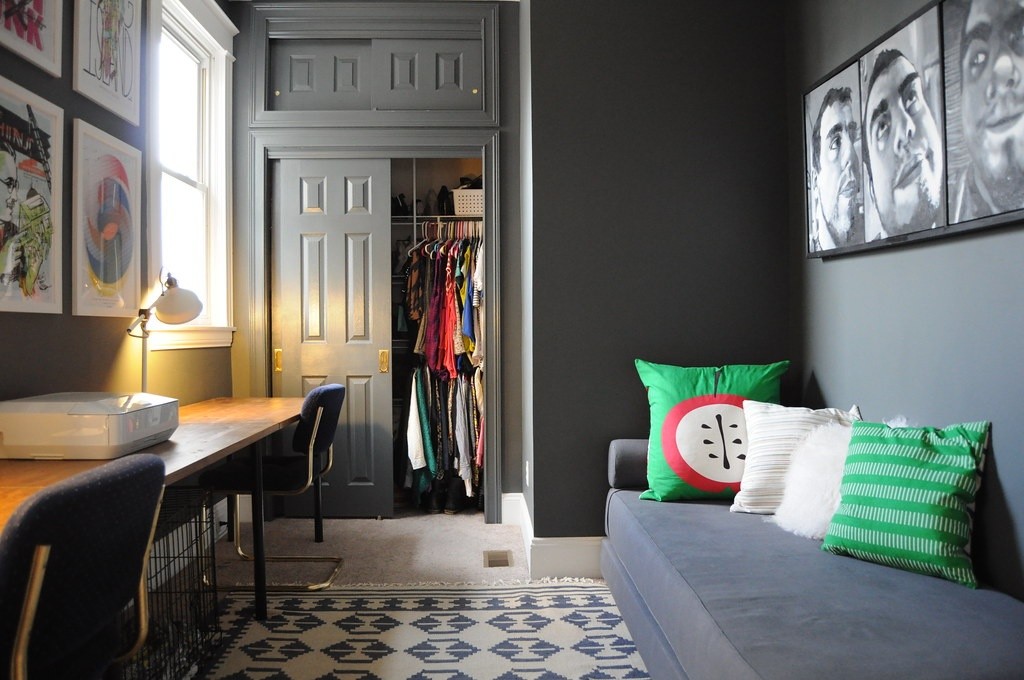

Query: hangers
407;221;481;260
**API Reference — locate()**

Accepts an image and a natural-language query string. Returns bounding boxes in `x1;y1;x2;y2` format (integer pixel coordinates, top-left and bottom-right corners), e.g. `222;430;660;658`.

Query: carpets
158;576;654;680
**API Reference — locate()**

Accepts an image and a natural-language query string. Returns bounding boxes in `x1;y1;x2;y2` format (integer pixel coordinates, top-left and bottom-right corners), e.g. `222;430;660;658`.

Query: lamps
126;272;204;395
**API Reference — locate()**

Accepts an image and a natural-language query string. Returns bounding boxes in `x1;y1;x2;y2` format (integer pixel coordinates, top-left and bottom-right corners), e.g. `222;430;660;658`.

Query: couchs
603;439;1023;680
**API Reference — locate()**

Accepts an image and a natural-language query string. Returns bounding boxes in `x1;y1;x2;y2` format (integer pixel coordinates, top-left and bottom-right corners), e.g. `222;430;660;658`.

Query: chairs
199;384;347;593
1;451;168;680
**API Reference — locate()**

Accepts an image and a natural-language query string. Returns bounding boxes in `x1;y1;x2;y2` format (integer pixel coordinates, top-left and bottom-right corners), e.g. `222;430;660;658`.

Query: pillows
775;420;905;539
729;396;863;515
823;419;989;590
634;360;792;503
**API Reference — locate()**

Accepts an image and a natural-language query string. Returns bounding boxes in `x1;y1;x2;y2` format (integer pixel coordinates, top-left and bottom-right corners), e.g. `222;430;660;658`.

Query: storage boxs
452;189;483;217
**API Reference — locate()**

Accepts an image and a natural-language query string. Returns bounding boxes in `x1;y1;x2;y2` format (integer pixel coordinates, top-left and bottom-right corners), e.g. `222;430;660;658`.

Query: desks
0;398;324;548
